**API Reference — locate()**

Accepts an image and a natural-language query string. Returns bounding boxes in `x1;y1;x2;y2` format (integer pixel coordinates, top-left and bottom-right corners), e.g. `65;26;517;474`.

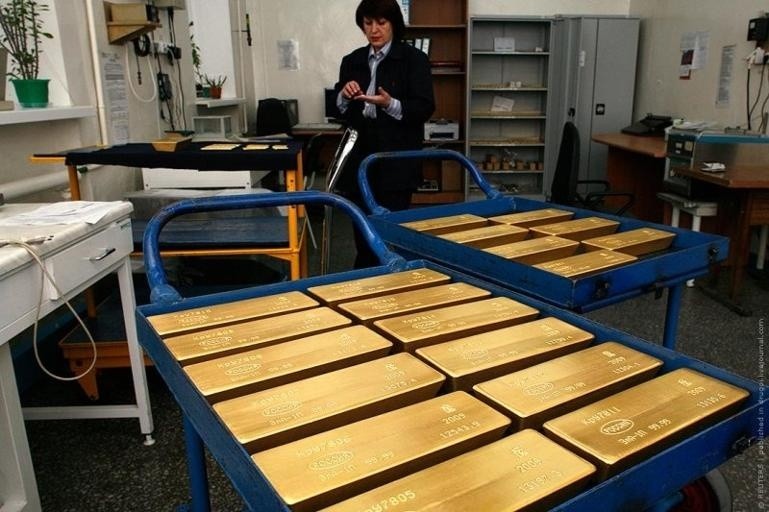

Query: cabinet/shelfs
404;0;468;205
0;262;44;330
468;18;553;202
46;216;135;301
0;106;102;202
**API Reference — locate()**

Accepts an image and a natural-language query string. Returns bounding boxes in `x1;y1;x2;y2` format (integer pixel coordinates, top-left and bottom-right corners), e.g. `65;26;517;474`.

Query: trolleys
134;189;769;512
358;147;730;349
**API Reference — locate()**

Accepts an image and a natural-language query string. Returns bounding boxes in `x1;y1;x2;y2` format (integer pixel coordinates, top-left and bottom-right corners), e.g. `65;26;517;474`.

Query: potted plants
0;1;54;109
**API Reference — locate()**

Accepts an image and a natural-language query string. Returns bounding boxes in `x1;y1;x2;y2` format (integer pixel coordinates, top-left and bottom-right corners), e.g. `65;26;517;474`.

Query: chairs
549;121;638;216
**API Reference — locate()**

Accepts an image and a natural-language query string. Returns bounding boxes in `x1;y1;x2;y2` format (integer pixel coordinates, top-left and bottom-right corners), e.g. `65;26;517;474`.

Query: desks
0;201;153;512
592;128;672;227
27;144;307;316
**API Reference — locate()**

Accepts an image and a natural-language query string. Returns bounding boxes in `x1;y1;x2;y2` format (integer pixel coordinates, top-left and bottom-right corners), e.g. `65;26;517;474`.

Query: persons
331;0;435;270
